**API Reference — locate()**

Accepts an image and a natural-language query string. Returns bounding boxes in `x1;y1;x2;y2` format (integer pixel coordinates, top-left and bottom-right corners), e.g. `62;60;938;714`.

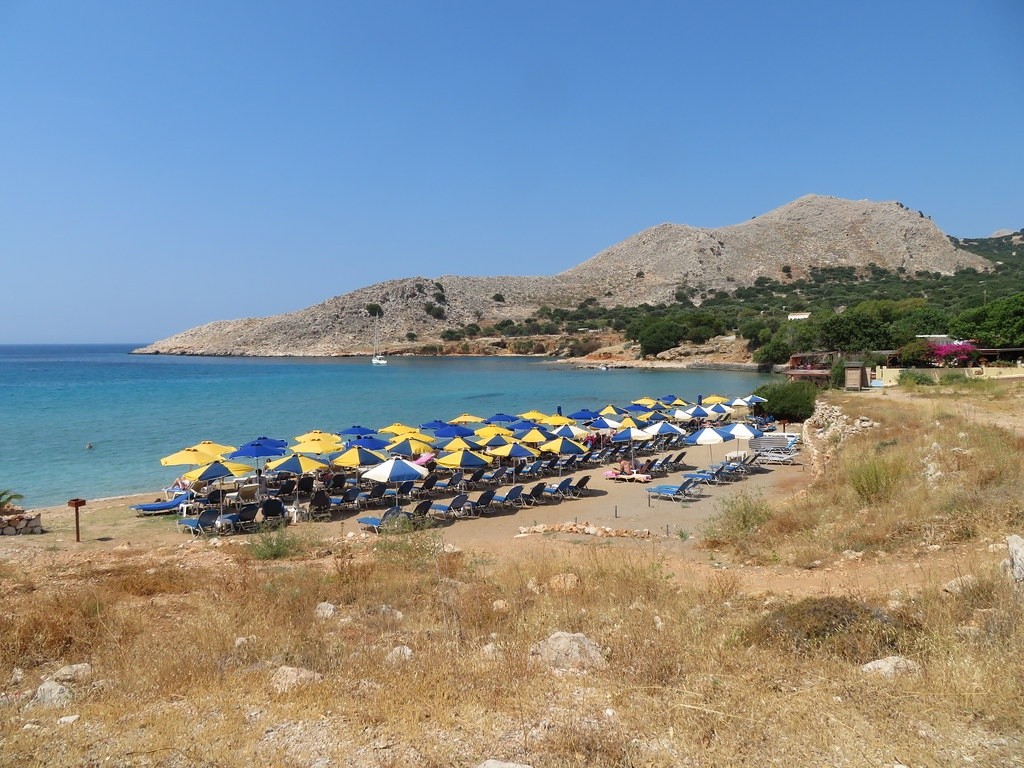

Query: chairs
130;412;801;538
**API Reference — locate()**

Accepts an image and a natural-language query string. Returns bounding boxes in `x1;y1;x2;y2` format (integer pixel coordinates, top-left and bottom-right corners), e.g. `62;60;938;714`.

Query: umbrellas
231;435;288;499
346;435;391;451
329;446;386;489
566;394;768;452
378;422;435;461
610;427;653;469
557;406;563;427
418;410;590;495
685;426;735;471
268;453;331;513
289;429;344;490
335;425;378;439
718;424;764;462
796;361;814;370
161;440;255;535
361;456;430;508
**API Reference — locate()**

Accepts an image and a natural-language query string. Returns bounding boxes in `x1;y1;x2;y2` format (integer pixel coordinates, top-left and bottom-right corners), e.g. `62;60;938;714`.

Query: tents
790;351;838;370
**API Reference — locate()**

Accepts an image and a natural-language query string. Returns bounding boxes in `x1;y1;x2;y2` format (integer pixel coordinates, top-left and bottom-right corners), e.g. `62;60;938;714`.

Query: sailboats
371;311;388;365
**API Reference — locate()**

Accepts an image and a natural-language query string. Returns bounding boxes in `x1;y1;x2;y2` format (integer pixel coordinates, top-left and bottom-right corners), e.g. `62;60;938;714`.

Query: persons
262;458;275;475
595;432;601;441
252;469;267;494
613;457;632;475
635;459;651;472
168;478;190;490
86;442;92;448
